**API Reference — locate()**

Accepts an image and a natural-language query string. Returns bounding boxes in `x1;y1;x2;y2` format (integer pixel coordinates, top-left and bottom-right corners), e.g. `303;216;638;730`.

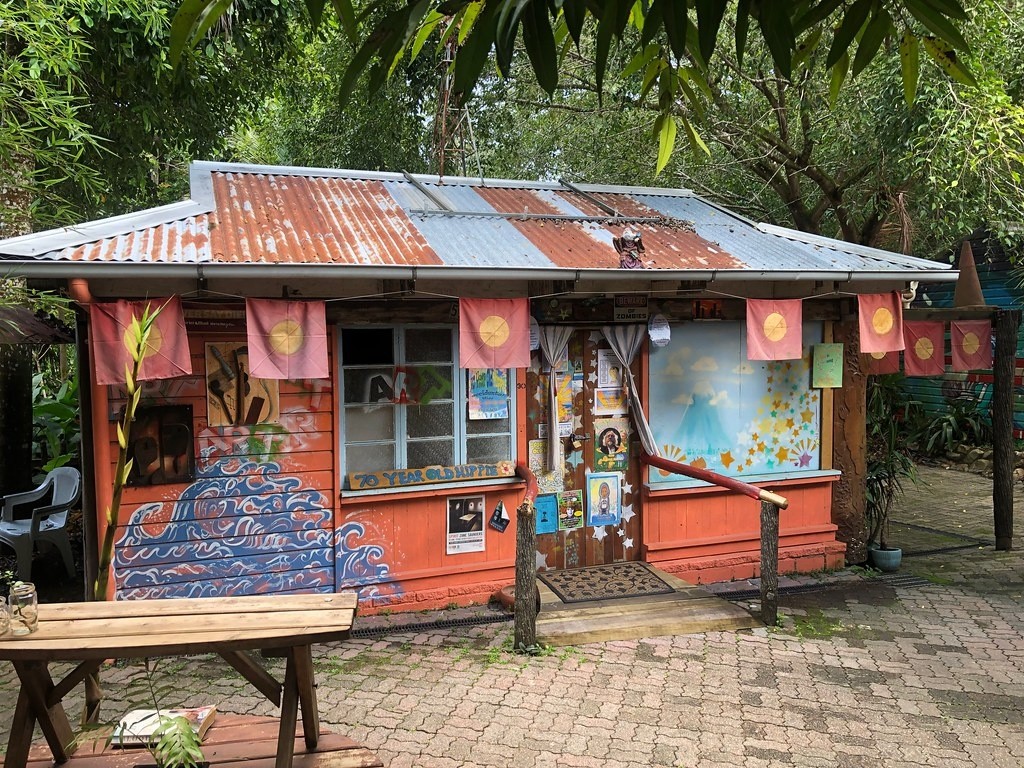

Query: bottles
0;596;9;635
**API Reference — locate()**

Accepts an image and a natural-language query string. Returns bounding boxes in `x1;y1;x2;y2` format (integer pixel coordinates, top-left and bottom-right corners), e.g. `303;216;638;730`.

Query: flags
87;294;193;386
457;296;531;368
901;319;947;377
858;349;900;375
744;297;804;361
244;296;331;380
949;321;993;372
857;291;907;353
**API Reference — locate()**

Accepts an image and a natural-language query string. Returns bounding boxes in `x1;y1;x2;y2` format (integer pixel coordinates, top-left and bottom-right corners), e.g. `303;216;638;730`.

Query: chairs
0;467;82;587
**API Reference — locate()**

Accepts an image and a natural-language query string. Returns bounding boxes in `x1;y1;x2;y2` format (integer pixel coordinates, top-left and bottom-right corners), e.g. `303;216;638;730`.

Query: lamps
647;307;670;347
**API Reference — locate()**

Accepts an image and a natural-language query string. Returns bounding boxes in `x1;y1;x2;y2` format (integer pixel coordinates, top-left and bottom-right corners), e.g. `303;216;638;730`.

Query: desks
0;593;358;768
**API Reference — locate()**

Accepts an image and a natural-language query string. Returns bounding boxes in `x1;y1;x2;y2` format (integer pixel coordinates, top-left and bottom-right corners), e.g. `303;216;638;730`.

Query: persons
613;228;647;270
598;430;620;455
